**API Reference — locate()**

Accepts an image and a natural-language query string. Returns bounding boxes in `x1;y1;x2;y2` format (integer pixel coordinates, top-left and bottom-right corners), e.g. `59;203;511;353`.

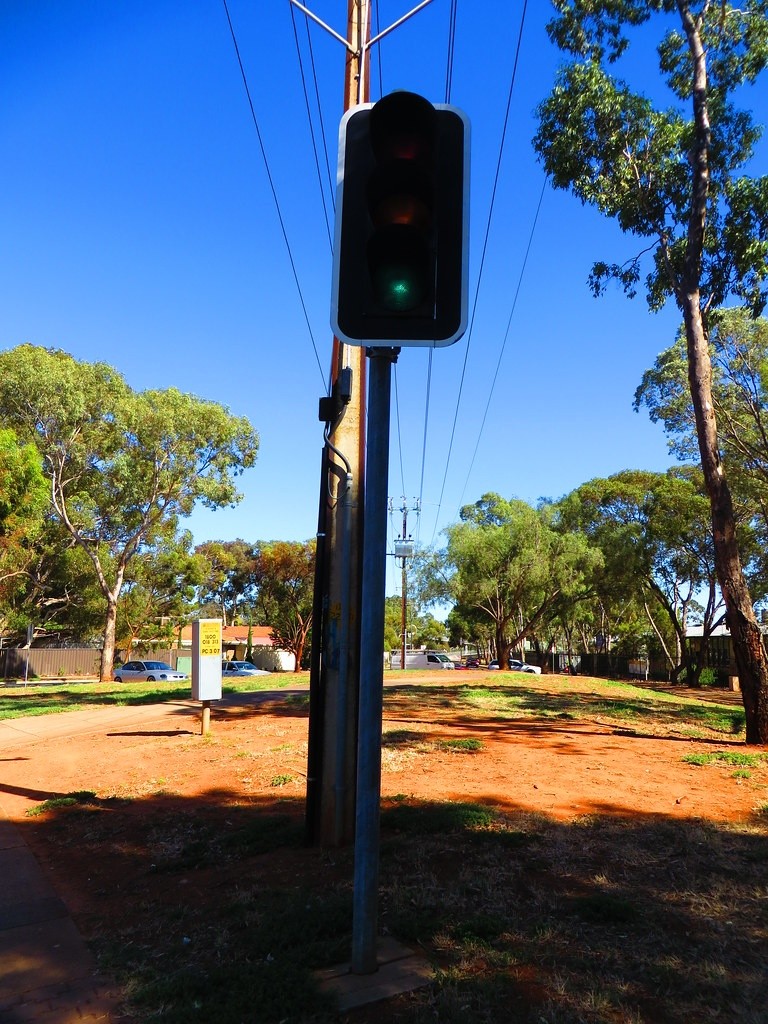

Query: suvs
487;659;541;675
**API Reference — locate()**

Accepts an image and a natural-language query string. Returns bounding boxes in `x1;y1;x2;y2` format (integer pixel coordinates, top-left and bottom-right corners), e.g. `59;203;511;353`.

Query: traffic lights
334;90;471;348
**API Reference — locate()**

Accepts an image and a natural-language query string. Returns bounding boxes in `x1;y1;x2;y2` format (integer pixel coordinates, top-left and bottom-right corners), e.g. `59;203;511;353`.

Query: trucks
390;651;455;670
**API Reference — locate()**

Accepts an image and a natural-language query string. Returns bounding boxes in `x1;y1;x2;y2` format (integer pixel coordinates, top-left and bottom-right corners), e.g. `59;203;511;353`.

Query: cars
222;661;271;677
112;660;189;684
465;658;480;668
